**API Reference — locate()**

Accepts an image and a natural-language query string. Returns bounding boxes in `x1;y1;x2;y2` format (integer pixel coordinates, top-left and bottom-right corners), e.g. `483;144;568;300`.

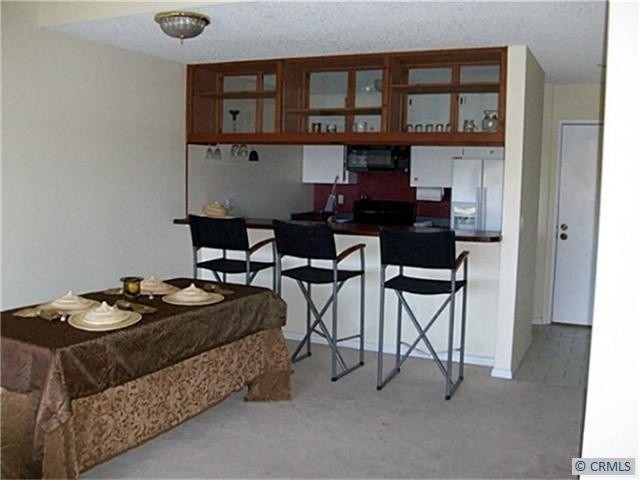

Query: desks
0;276;294;480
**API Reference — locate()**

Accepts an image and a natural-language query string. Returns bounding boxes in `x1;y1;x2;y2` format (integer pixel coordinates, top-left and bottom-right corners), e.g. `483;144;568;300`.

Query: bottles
483;109;498;133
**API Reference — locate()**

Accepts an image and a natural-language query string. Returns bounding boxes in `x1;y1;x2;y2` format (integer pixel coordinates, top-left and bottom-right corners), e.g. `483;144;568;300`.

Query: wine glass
205;141;249;161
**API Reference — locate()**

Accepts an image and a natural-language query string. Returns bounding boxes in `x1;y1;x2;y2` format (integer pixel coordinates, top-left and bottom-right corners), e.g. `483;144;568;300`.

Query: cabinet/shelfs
184;44;508;189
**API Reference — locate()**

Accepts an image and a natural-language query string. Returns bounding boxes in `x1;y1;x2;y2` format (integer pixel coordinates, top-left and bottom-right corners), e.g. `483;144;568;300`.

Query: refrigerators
450;157;505;233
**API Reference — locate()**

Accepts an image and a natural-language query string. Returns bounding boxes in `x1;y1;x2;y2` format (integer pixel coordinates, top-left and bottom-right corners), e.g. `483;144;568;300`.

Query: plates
67;307;142;331
140;281;181;296
162;288;225;307
37;298;101;316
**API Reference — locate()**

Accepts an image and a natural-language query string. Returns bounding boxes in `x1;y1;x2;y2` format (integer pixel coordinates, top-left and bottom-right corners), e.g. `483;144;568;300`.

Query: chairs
188;213;285;293
272;218;366;381
377;227;470;401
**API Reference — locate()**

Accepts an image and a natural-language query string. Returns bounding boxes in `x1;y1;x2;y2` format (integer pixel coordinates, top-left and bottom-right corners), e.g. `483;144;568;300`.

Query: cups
407;123;451;134
311;123;321;133
354;120;366;133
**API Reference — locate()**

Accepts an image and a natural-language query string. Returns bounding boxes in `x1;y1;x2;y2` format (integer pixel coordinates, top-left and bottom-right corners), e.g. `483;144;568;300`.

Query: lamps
154;11;211;39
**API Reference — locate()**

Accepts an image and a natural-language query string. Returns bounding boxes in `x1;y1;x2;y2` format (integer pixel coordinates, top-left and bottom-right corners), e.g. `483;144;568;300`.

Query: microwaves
348;144;410;172
